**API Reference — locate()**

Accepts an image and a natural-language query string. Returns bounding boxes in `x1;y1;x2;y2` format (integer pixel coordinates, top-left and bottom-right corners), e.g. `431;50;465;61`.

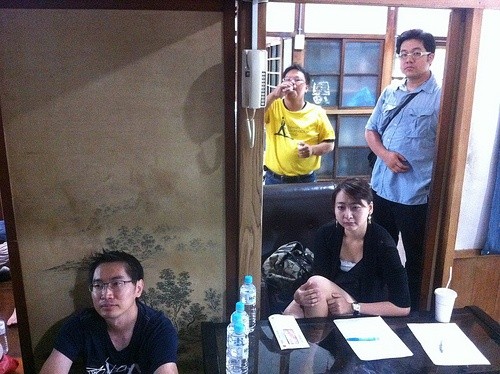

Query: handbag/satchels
261;241;324;317
366;151;377;168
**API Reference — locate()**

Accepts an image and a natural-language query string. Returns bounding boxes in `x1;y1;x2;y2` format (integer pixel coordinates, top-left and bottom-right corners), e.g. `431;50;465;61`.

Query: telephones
242;49;268;109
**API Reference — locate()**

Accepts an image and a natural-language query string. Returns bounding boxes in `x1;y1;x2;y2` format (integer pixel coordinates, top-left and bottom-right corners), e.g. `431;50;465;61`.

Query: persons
365;29;446;312
282;177;412;319
262;63;336;186
39;250;178;374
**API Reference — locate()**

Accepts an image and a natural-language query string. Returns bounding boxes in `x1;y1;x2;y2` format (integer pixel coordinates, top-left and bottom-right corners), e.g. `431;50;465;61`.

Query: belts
266;168;314;182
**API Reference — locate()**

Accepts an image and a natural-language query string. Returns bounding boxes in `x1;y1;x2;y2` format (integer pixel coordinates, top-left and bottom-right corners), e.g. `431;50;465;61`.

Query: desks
201;306;500;374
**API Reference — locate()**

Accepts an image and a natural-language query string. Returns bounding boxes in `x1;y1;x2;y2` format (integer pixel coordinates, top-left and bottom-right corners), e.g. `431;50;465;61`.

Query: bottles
226;314;248;374
229;323;248;374
230;302;249;336
239;275;257;333
0;318;9;355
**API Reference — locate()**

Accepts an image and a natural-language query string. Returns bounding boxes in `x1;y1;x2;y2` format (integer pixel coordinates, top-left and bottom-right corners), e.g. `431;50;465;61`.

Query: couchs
262;181;338;264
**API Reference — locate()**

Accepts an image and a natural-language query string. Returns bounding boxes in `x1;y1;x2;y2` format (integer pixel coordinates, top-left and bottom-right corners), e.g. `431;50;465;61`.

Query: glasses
396;50;432;61
282;77;307;85
88;279;134;293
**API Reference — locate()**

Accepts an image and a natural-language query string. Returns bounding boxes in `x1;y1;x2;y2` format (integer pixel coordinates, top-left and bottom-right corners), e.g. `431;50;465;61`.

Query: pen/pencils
346;337;380;341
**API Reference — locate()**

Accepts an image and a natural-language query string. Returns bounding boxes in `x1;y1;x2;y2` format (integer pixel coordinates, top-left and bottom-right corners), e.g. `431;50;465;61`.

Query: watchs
351;301;360;318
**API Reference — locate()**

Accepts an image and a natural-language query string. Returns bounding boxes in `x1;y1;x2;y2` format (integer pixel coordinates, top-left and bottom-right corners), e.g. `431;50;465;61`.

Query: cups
434;288;458;323
